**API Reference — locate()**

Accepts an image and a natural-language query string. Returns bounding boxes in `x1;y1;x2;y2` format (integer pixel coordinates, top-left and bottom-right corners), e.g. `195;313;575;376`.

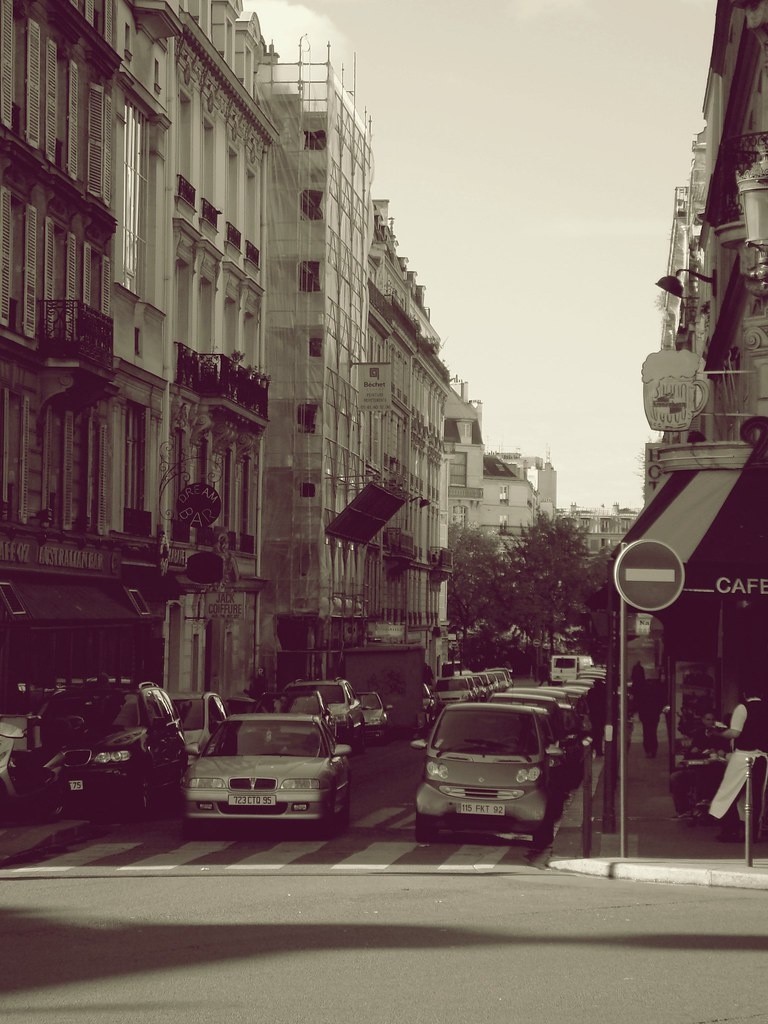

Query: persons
707;679;768;843
537;664;551;686
587;660;730;819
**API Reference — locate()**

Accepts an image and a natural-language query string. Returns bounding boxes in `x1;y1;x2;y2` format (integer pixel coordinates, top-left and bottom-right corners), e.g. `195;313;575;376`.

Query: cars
177;710;352;839
411;652;607;851
248;686;338;751
355;690;396;746
166;689;229;770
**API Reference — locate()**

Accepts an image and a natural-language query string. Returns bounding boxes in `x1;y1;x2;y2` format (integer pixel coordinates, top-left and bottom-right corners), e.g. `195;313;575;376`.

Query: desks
680;757;728;819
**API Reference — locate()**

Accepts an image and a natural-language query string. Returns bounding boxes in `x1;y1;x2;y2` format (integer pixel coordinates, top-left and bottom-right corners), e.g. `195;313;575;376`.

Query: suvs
22;677;189;826
280;675;365;745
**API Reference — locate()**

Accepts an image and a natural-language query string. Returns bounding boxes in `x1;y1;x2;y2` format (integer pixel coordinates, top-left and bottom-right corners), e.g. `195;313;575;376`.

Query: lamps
654;268;717;300
428;626;440;637
410;496;430;508
29;507;54;547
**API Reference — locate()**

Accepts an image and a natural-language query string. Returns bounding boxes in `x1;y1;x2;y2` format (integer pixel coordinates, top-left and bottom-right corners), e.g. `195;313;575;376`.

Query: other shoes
716;832;742;844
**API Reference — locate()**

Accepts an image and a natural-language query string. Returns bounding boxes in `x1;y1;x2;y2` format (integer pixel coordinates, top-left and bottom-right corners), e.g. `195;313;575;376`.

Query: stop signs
612;537;687;614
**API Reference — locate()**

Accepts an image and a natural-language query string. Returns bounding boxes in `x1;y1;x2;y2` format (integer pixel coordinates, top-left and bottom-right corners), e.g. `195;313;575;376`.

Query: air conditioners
430;546;454;570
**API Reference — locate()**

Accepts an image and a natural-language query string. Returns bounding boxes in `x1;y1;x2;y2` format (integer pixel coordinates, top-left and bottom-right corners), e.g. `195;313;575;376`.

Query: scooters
0;716;71;823
